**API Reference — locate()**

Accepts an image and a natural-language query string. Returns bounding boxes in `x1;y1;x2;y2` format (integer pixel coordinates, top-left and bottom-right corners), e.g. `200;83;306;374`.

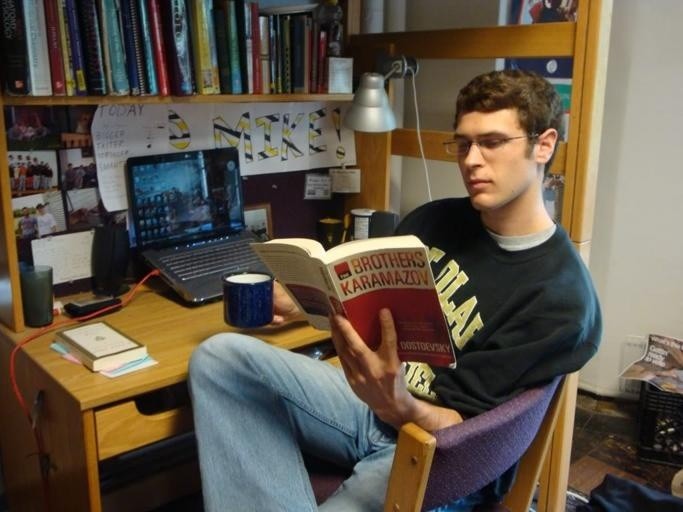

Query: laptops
126;146;275;305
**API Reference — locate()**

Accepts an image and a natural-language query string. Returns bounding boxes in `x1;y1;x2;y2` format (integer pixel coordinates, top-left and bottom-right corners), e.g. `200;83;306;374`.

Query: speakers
368;211;399;239
90;227;129;297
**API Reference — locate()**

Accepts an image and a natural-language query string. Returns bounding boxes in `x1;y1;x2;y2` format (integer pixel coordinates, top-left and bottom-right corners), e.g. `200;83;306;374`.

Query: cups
317;217;343;252
19;264;54;328
221;270;274;329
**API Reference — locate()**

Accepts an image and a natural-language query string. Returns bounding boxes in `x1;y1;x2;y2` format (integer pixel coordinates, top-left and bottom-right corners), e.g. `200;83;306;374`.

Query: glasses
442;134;539;155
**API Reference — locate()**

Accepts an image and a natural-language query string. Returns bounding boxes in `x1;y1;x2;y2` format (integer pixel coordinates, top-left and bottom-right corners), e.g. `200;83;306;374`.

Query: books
247;233;457;370
0;0;354;98
53;321;148;373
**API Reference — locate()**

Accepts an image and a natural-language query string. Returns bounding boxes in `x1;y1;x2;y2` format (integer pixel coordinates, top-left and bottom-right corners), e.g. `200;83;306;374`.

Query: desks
0;272;342;512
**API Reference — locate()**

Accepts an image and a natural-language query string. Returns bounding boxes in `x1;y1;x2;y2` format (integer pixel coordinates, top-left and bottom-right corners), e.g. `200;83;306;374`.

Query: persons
185;69;602;512
4;155;53;191
16;203;57;238
64;162;97;188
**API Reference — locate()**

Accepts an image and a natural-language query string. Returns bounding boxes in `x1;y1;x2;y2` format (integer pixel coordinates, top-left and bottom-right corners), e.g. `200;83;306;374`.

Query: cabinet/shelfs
0;0;392;329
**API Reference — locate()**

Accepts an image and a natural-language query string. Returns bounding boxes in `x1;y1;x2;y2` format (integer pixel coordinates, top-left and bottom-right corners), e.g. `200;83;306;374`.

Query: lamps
341;54;418;134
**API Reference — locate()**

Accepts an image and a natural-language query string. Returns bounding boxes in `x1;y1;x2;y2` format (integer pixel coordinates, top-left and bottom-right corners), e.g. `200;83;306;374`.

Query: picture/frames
7;150;60;196
65;187;113;230
243;200;274;240
11;188;70;242
58;145;98;189
495;0;576;85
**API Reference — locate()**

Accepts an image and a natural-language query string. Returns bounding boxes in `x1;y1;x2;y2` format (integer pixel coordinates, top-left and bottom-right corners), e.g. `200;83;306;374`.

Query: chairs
311;372;580;512
